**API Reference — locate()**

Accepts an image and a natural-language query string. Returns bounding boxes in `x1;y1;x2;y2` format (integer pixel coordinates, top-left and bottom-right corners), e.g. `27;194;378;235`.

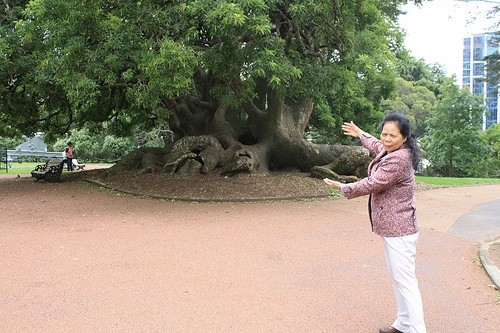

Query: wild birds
12;174;21;181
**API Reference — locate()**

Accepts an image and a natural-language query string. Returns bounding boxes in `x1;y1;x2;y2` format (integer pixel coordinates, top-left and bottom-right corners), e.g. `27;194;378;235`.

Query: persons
323;113;426;333
66;142;73;172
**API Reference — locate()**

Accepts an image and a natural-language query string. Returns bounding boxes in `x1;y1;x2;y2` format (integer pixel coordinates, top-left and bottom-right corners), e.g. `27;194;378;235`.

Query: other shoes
379;326;400;333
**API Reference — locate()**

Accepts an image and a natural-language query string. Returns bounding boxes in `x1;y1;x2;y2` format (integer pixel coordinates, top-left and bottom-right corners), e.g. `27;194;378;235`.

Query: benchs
31;158;68;181
72;159;85;169
